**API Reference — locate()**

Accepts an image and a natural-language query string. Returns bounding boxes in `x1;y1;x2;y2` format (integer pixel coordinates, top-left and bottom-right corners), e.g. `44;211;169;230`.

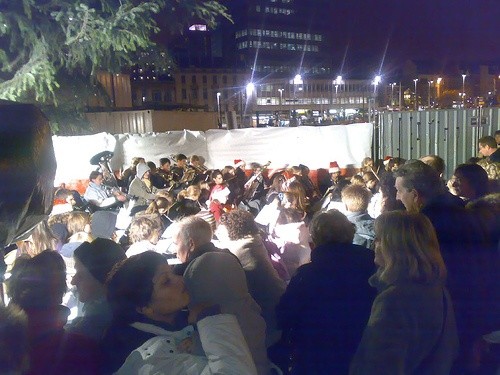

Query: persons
0;130;500;375
